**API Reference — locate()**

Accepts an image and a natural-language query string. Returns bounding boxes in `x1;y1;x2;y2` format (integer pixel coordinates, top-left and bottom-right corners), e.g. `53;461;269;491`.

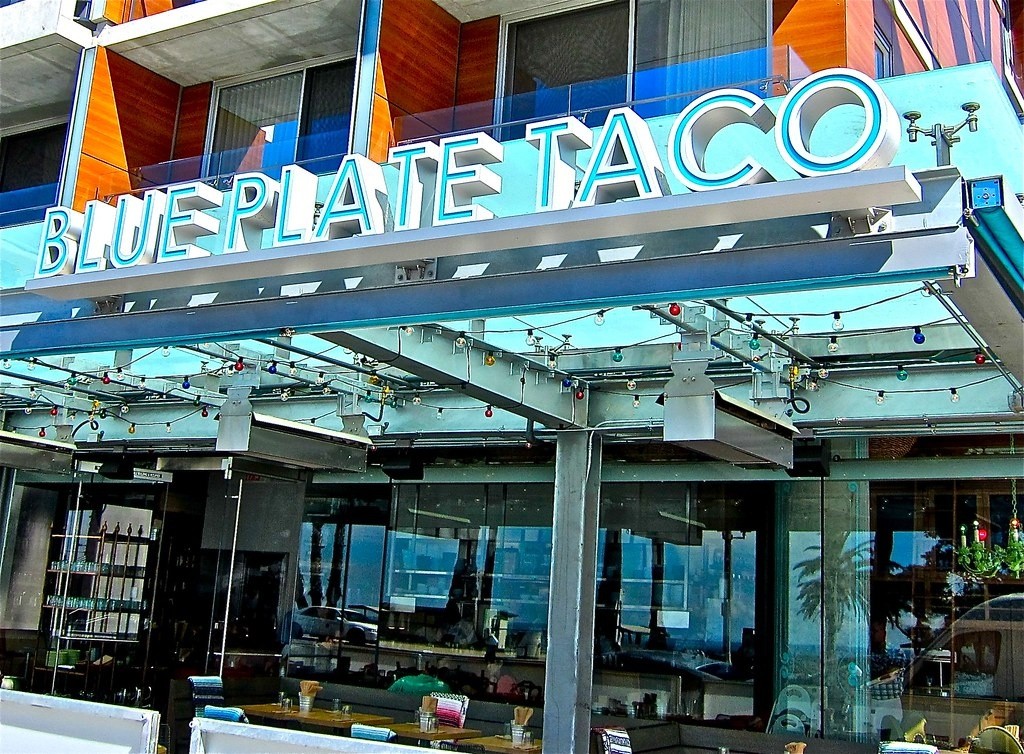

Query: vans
845;593;1024;741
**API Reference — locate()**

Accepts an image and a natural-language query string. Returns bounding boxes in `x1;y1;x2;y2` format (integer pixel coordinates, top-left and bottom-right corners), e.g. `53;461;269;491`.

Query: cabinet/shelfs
43;533;157;692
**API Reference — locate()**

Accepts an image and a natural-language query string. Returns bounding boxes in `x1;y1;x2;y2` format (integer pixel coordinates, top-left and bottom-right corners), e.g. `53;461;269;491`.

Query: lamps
951;433;1024;583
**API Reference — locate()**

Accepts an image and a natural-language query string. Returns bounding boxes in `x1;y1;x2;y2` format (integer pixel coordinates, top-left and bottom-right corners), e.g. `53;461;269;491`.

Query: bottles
523;731;534;747
429;717;439;732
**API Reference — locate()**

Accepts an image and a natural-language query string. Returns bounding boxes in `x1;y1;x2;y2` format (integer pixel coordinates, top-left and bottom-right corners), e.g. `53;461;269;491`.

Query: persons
386;661;452;698
840;613;906;732
414;611;478;647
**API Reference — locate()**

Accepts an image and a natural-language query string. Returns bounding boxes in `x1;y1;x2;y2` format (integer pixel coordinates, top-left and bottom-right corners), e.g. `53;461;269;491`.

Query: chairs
351;723;398;744
416;692;470;751
853;667;906;732
765;683;819;737
879;708;1024;754
204;704;250;724
189;676;224;717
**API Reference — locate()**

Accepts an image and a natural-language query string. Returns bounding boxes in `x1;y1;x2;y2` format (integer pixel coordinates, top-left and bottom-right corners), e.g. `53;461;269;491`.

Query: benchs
4;629;47;678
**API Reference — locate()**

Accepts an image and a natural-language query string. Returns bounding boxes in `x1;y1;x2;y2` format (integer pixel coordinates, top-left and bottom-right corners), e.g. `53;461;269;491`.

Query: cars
291;606;379;644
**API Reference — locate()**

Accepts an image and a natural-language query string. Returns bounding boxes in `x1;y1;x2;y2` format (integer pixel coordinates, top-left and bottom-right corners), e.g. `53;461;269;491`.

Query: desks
235;703;395;736
378;640;518;670
375;722;483;749
705;682;828;720
457;735;543;754
35;666;86;693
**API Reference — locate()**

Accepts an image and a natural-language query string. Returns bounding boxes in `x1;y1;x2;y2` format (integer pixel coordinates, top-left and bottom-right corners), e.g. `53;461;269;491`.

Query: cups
927;678;932;687
420;712;434;731
332;699;341;711
511;724;526;745
657;702;667;719
299;692;314;712
114;688;126;705
281;699;293;712
341;706;352;720
279;692;287;703
627;706;638;718
